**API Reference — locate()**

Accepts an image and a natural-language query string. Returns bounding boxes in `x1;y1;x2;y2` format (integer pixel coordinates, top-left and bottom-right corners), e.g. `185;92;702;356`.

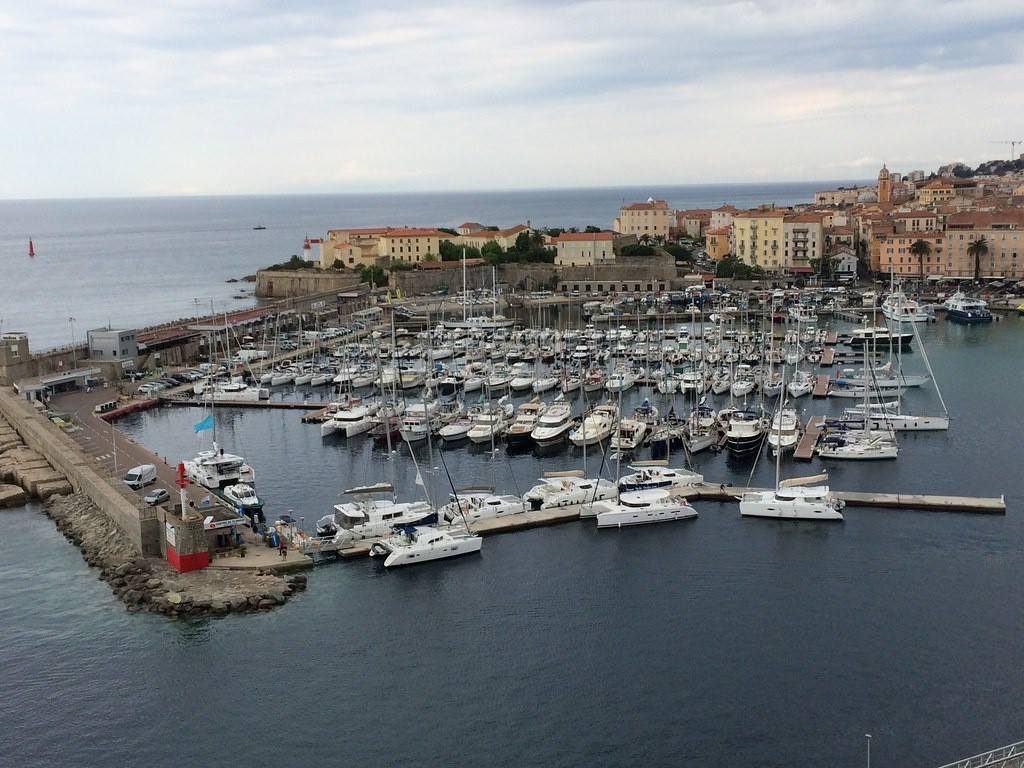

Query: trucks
302;331;323;343
200;363;226;375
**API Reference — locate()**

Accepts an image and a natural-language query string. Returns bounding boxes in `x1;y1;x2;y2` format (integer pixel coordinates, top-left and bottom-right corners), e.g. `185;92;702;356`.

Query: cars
172;374;191;382
324;332;336;338
146;378;180;390
180;372;198;381
138;384;158;394
875;280;883;284
188;371;204;378
341;324;356;331
241;342;257;349
144;489;170;506
338;327;347;333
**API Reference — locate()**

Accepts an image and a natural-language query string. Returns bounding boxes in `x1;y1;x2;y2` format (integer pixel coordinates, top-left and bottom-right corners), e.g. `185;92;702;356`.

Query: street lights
287;510;293;542
300;516;306;553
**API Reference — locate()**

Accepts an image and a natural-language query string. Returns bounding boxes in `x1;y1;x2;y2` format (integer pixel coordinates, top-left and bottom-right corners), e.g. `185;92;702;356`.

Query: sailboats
179;249;952;568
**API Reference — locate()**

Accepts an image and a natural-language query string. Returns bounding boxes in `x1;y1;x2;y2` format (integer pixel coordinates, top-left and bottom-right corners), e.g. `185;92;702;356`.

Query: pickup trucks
539;290;551;295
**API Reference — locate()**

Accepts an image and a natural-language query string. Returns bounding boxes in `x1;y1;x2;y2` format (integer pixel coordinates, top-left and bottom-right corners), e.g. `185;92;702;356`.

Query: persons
161;371;167;378
339;318;340;324
720;484;724;492
563;290;569;298
283;544;287;561
804;423;807;434
253;524;258;536
279;538;284;556
87;387;93;393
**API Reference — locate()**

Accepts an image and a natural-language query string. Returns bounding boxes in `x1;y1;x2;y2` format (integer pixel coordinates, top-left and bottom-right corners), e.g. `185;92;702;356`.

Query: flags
195;413;214;433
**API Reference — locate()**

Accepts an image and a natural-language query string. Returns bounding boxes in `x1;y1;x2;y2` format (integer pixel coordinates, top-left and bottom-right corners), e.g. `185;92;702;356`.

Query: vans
233;349;258;361
123;463;157;490
256;351;268;358
327;328;344;336
273;333;288;341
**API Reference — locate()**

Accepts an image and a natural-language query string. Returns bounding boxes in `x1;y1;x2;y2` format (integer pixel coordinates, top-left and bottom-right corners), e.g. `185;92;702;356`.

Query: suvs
281;341;297;350
124;369;145;379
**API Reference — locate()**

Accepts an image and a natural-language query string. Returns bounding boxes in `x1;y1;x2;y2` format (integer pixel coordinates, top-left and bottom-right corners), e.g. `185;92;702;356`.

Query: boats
1015;304;1024;312
944;296;994;324
882;287;936;322
